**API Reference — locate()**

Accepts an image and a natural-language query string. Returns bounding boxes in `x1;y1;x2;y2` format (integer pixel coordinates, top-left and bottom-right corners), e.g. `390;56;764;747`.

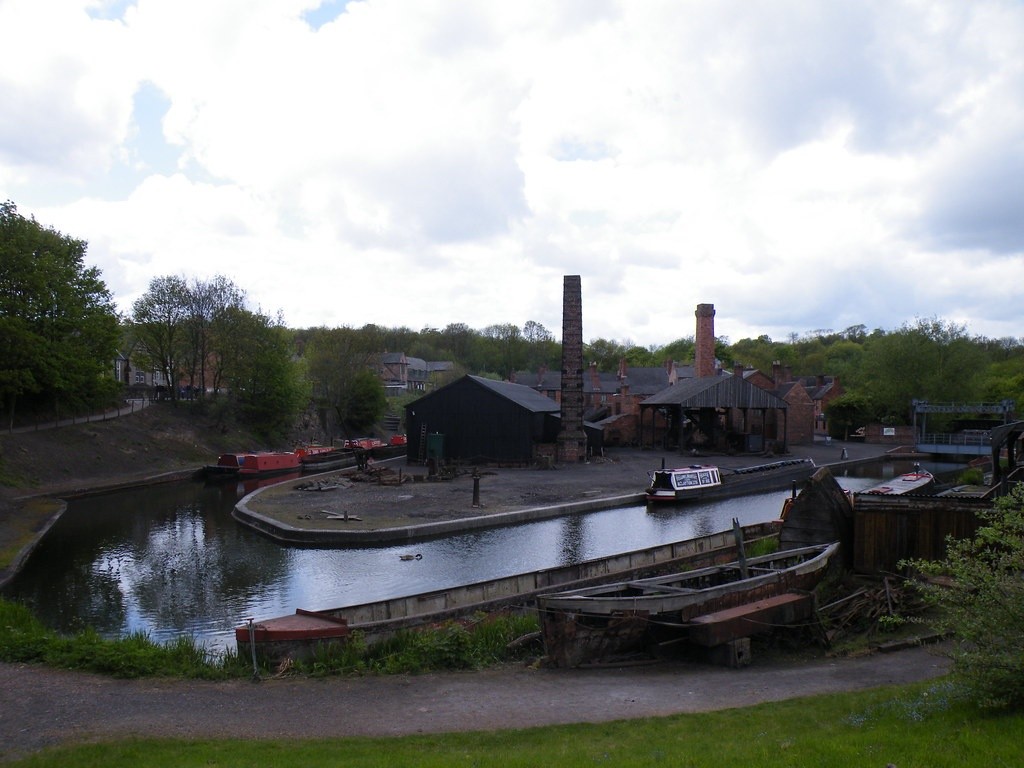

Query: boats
644;456;724;501
202;433;407;473
537;516;841;672
859;461;935;495
